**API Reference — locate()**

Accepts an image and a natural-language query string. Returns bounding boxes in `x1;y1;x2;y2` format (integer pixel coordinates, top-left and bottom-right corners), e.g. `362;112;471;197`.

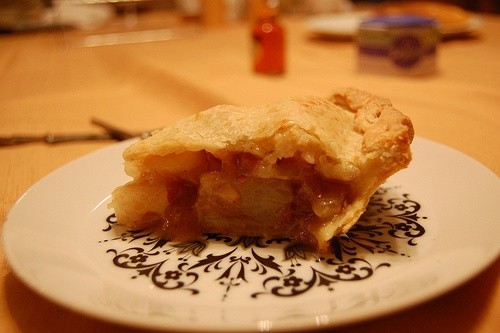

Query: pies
107;85;414;263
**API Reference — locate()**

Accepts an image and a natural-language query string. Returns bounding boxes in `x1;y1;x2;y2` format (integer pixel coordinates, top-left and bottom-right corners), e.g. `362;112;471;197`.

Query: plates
3;132;500;331
307;12;482;38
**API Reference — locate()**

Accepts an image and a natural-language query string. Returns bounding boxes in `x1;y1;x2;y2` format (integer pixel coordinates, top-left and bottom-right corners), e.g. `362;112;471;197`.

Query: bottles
252;2;286;74
